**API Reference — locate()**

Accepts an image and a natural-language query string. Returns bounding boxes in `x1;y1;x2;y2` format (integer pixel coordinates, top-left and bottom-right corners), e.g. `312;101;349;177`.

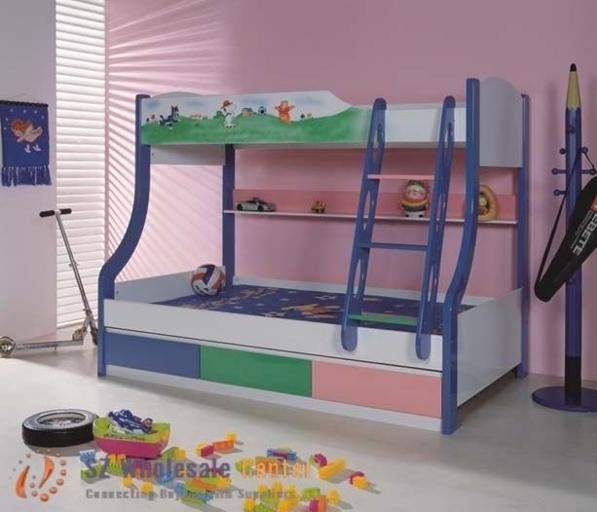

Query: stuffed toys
79;408;369;512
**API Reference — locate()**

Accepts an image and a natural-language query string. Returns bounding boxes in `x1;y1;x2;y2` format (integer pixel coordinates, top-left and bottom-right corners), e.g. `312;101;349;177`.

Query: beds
98;76;530;435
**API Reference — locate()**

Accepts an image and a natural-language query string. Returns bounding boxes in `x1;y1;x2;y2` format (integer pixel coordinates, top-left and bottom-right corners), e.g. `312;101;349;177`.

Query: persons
399;181;430;216
